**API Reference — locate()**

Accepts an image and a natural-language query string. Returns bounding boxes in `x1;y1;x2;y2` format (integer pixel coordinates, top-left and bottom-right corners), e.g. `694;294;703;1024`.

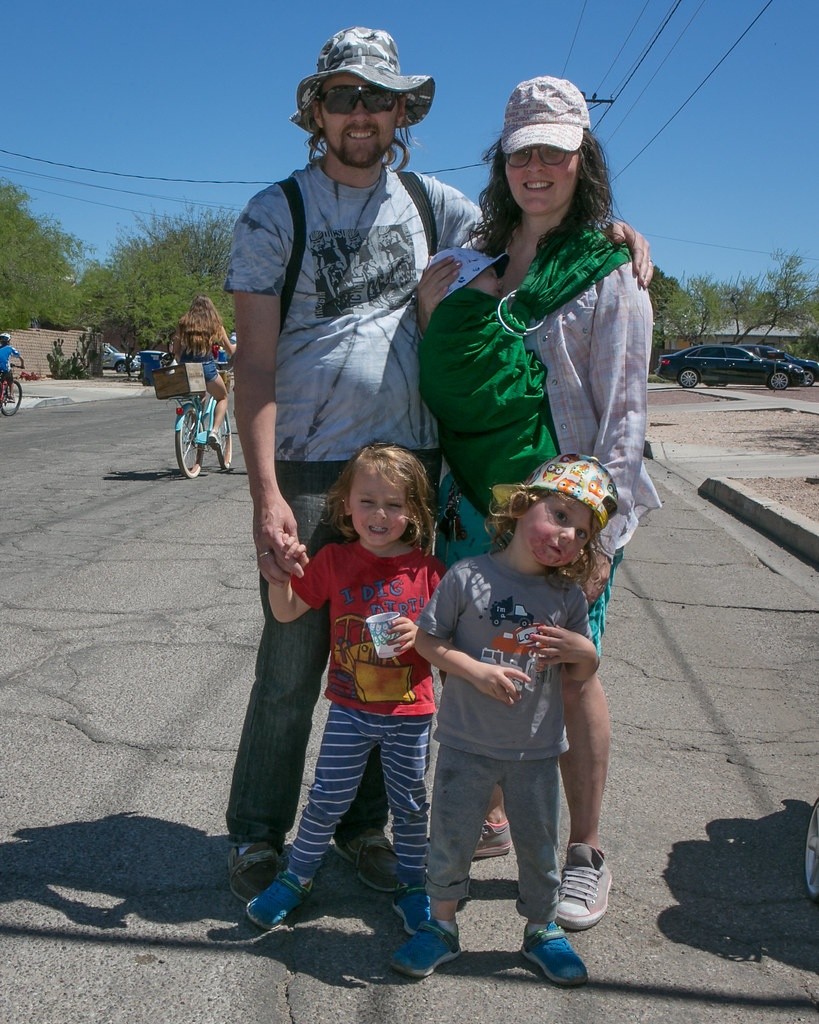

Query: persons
173;295;236;450
418;247;557;520
223;29;656;894
416;74;662;931
389;453;620;987
244;442;449;935
0;332;24;403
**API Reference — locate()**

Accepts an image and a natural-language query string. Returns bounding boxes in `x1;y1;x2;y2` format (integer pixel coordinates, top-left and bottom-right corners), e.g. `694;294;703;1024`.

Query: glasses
314;83;403;115
504;145;568;169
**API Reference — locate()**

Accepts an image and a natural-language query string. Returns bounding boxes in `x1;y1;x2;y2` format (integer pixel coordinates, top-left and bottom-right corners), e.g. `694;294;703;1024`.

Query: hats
289;27;434;129
427;247;511;303
501;74;590;154
494;451;619;532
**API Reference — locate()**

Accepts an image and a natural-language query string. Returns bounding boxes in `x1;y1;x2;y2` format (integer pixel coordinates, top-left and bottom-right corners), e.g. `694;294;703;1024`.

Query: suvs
103;343;141;373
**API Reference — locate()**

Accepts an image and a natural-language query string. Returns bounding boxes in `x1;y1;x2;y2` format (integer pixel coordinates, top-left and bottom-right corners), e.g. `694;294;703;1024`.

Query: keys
439;507;460;542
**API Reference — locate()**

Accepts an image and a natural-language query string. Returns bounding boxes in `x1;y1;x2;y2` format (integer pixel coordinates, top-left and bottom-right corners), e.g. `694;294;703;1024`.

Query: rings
650;259;654;265
259;551;269;557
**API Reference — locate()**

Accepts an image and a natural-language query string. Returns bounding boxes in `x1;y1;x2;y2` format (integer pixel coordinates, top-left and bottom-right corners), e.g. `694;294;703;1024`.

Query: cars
737;344;819;386
655;345;806;390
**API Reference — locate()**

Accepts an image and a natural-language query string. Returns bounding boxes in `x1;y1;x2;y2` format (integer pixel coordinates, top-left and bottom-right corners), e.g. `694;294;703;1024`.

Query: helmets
0;332;11;344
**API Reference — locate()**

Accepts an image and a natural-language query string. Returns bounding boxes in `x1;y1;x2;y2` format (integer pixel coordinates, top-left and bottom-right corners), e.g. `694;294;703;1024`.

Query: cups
365;611;402;659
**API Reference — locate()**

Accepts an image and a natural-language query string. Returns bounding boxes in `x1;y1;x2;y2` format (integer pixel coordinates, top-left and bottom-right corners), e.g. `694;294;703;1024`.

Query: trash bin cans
218;350;227;362
139;350;165;386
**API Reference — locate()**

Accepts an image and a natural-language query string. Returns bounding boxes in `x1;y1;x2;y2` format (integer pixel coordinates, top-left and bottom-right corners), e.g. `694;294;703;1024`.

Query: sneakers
390;882;432;936
471;819;512;859
554;842;612;931
387;919;464;979
244;867;314;931
523;920;587;989
227;847;279;901
335;834;402;891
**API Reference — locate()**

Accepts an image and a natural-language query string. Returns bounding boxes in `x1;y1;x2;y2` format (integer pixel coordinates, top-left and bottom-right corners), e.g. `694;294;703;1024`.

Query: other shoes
208;429;220;451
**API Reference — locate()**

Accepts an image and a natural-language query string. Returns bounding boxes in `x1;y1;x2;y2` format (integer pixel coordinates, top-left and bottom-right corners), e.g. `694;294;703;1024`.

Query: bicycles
171;360;235;478
0;363;24;416
